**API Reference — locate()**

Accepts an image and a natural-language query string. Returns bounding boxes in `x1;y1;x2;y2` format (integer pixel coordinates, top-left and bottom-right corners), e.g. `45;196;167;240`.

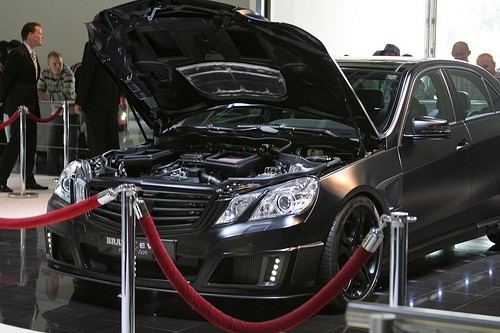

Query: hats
380;44;400;55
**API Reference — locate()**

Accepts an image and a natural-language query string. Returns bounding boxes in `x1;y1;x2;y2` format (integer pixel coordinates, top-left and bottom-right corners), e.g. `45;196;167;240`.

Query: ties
31;52;38;79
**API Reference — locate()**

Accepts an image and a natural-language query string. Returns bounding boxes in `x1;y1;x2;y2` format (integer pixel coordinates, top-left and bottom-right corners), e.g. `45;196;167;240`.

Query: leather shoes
26;182;48;189
0;184;13;192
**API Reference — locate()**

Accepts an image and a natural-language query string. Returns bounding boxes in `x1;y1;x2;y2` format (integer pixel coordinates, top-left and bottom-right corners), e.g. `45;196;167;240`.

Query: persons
476;53;500;81
372;41;471;63
0;14;124;176
0;22;48;193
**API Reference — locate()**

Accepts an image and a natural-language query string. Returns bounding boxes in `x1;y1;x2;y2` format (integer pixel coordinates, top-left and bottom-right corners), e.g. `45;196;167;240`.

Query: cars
41;0;500;320
